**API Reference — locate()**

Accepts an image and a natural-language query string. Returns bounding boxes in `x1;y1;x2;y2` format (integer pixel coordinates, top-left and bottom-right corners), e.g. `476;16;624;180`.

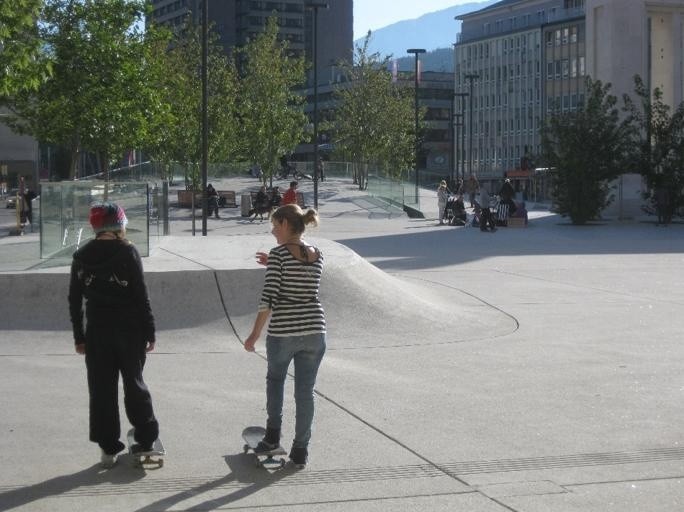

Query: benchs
251;191;310;222
178;190;240;208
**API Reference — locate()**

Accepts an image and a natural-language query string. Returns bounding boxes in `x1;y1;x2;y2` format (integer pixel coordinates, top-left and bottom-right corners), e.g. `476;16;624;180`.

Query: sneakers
138;440;155;451
100;451;118;466
258;433;281;449
289;448;308;469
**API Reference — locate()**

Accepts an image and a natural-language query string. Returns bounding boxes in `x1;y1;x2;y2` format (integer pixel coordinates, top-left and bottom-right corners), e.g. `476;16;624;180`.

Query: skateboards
241;424;290;468
127;426;165;469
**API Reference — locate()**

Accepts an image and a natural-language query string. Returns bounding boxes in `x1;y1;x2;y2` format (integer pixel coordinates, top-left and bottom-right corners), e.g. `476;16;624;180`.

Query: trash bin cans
240;194;252;217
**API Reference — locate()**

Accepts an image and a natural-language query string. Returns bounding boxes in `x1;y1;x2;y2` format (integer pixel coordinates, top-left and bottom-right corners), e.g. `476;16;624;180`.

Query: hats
90;202;127;234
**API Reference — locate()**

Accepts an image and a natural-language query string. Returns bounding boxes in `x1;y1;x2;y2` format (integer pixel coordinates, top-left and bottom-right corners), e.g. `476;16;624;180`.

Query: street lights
453;75;479;184
306;1;329;211
406;48;426;204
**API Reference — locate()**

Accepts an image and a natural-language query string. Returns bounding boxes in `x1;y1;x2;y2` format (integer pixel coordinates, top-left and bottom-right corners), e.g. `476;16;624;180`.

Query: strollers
444;196;467;225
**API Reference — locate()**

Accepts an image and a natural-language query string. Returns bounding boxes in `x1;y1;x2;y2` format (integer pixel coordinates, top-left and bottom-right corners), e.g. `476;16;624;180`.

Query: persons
254;184;271;210
24;188;36;224
68;201;161;466
280;156;290;179
272;185;282;204
243;203;328;470
207;184;220;218
281;180;300;205
437;173;529;232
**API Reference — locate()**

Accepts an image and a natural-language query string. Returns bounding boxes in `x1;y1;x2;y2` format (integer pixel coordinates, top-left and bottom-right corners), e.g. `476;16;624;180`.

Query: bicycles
274;166;305;180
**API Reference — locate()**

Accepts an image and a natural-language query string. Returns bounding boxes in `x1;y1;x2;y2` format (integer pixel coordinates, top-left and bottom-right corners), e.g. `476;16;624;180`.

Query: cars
6;188;28;209
